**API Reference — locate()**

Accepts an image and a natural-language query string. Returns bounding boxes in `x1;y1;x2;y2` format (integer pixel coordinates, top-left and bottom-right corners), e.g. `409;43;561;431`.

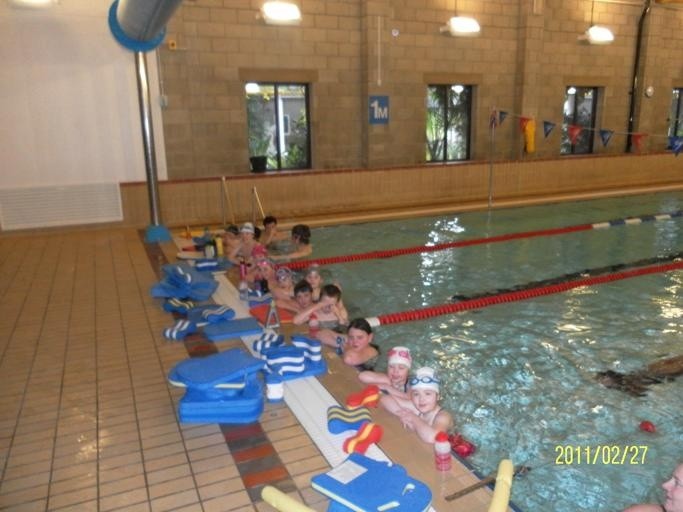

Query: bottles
216;235;224;257
240;258;247;279
433;432;453;472
308;312;320;337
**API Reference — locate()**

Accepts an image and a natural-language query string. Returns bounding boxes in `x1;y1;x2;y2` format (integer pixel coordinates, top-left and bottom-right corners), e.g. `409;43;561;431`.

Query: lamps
439;15;482;37
255;1;304;26
578;26;615;46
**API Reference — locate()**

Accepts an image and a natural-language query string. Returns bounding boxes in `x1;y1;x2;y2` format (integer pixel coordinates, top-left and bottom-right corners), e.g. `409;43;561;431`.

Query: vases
250;155;267;173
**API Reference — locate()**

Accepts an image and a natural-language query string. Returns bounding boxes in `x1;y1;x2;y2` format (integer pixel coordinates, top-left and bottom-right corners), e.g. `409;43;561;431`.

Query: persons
220;216;455;446
621;460;683;512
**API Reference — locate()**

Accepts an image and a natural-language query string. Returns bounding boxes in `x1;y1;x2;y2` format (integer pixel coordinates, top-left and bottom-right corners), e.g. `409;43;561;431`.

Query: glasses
256;261;267;266
386;350;409;359
239;226;253;230
409;377;434;384
335;336;343;356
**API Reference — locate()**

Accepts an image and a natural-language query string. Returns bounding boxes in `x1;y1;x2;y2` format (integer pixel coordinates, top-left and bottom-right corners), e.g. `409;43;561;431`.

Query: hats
276;267;290;283
305;263;321;277
389;346;411;367
240;223;254;234
412;367;439;392
251;245;266;256
